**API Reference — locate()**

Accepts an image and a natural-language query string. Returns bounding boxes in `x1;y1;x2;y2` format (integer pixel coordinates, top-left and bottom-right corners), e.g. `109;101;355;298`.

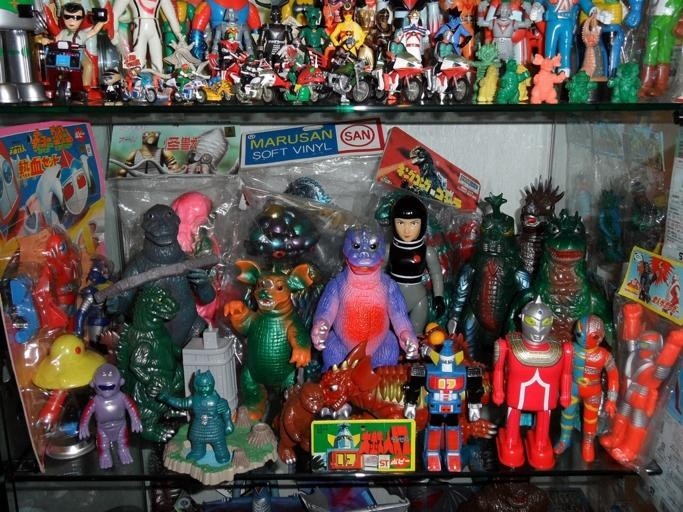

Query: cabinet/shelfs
1;96;683;494
